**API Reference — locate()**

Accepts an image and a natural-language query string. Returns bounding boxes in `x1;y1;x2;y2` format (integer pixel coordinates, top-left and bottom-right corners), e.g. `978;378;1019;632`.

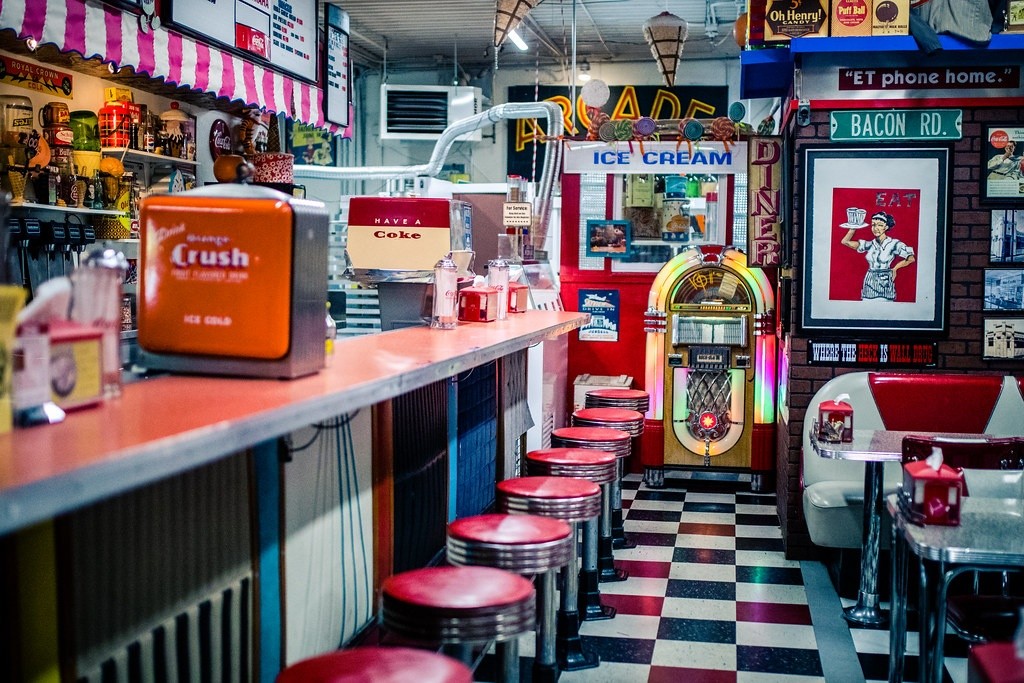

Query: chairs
900;434;1024;646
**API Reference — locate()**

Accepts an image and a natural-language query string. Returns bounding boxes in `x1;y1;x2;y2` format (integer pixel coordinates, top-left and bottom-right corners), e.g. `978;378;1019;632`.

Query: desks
881;492;1024;683
809;428;1024;625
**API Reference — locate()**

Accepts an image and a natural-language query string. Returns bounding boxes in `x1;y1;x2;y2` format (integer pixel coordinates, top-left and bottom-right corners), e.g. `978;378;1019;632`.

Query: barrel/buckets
97;100;130;148
43;148;73;177
72;151;102;177
686;175;717;197
665;176;688;198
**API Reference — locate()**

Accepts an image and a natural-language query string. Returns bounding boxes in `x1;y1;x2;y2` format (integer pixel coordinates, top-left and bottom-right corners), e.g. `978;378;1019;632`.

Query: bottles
92;169;103;209
131;123;139;148
0;95;100;151
662;198;690;241
488;259;509;320
144;110;167;155
705;192;718;242
75;248;129;400
430;259;457;329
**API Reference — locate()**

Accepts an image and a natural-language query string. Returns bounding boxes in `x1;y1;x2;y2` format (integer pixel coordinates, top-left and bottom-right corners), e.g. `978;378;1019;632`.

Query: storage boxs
104;87;132;102
128;104;147;134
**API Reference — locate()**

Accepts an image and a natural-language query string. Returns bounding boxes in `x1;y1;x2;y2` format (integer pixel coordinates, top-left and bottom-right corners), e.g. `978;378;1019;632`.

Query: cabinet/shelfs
7;147;201;242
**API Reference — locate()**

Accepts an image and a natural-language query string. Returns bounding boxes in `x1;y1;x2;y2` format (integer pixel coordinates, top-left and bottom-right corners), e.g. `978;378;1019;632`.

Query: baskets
93;218;132;238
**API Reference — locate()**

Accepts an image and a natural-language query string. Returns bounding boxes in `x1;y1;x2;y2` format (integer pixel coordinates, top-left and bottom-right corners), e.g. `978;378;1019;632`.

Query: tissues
14;276;105;415
902;446;961;525
458;275;498;322
818;393;853;444
508;268;528;313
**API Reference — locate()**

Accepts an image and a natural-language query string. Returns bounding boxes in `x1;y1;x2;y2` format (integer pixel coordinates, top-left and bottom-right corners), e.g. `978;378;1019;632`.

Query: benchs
797;371;1024;598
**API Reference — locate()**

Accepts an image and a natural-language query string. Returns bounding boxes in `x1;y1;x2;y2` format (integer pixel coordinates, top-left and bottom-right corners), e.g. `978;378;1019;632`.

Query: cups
253;152;294;183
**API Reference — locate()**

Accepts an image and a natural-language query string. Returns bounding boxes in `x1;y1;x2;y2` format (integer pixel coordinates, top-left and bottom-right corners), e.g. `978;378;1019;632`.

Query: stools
276;388;652;683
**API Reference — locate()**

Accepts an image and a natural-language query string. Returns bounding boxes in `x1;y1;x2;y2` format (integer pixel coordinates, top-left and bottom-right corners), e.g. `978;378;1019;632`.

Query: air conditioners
380;83;483;141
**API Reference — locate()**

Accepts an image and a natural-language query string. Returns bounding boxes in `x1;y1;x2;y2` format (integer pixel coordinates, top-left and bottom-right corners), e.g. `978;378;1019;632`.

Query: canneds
42;102;72;144
0;95;33;168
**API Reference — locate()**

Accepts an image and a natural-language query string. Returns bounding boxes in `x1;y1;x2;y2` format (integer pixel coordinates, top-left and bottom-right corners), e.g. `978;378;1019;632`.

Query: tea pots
213;155;255;183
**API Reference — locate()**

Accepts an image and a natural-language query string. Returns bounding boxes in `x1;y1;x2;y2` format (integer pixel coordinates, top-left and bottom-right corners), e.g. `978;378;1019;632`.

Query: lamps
25;35;43;54
108;61;122;74
578;57;591;81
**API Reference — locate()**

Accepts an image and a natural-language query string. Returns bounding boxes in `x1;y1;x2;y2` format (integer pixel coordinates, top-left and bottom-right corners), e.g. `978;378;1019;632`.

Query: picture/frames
587;219;632;257
797;143;955;339
979;120;1024;362
